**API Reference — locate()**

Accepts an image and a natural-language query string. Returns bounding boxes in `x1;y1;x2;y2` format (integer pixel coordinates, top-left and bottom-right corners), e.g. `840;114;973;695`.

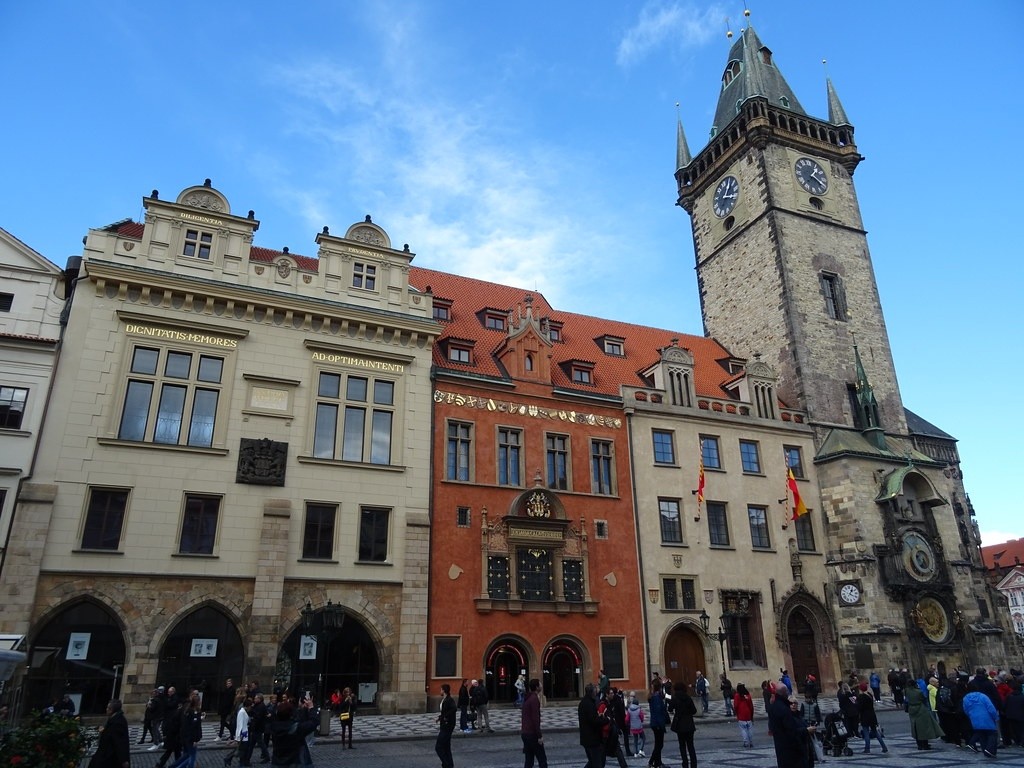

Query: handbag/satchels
730;688;737;699
341;713;349;720
665;694;671;700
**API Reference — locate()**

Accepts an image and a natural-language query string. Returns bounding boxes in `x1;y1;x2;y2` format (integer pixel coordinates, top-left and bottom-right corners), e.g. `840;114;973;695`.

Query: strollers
813;702;859;757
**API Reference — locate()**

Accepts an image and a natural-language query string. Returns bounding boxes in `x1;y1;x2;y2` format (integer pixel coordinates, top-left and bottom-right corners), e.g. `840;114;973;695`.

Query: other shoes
639;750;646;757
725;714;731;717
743;744;748;747
626;752;634;756
487;729;495;733
213;737;224;743
881;749;888;754
479;728;483;732
634;753;642;758
984;750;997;758
862;751;871;755
957;745;962;749
968;745;978;754
147;742;165;750
513;702;516;707
472;726;478;730
750;744;754;747
657;763;670;768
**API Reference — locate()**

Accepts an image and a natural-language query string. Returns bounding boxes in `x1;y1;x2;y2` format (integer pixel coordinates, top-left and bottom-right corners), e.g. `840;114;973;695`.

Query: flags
789;469;808;520
697;454;704;502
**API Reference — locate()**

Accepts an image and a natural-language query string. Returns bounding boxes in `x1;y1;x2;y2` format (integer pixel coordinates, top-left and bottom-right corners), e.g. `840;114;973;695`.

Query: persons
513;675;526;707
520;679;549;768
578;670;697;768
86;699;131;768
214;679;321;768
719;674;736;716
332;687;357;750
836;681;888;754
46;695;75;717
435;685;457;768
762;670;822;768
694;671;710;712
457;679;494;733
869;670;881;702
886;664;1024;757
734;682;754;747
137;686;206;768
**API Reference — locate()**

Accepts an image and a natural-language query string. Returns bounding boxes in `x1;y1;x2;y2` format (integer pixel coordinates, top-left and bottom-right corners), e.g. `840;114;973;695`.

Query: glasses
609;693;612;694
790;702;797;705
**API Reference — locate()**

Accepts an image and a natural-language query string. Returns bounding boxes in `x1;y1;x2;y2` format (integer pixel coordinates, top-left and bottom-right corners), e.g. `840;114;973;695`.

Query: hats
471;679;477;685
859;683;868;692
808;675;816;682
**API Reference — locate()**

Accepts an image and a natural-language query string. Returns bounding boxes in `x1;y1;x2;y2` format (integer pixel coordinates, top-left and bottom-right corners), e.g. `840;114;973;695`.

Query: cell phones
814;722;817;727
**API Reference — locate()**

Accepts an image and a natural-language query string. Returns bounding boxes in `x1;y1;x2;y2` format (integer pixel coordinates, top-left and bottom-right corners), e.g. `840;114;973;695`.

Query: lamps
839;582;861;606
710;174;740;218
793;154;828;195
